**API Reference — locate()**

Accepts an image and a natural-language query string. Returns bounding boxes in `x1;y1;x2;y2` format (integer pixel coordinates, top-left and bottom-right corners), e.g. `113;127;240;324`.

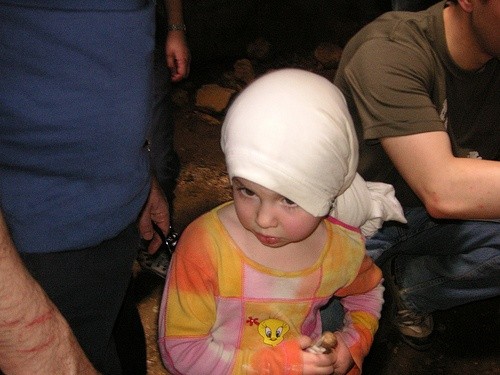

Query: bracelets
166;23;187;33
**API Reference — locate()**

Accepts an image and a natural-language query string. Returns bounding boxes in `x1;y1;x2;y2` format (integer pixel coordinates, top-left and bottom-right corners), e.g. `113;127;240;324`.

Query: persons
320;0;500;350
158;67;407;375
136;0;191;277
0;0;170;375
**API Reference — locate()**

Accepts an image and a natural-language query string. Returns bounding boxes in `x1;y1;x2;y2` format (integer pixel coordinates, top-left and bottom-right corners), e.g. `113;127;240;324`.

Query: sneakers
380;254;437;351
